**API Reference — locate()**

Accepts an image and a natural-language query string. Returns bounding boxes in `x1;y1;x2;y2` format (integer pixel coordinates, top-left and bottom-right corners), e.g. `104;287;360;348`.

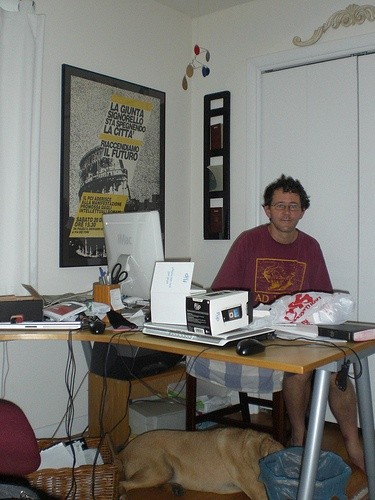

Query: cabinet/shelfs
86;364;185;449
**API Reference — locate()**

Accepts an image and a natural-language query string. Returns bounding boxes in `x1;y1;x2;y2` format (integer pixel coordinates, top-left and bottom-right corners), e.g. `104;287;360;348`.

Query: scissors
112;263;128;284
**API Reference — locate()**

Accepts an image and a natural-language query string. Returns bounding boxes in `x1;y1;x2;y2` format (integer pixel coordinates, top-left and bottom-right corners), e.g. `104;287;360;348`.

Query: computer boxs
127;397;186;437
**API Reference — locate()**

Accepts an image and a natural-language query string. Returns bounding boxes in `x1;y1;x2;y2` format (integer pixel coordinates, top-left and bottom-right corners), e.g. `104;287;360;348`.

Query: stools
186;367;291;447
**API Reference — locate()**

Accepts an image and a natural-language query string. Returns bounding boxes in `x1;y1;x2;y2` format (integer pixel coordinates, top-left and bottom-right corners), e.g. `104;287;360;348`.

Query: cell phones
221;305;242;322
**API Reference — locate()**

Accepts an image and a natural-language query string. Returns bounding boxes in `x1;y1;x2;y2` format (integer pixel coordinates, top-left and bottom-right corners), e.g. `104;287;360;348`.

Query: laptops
142;320;276;347
0;321;83;330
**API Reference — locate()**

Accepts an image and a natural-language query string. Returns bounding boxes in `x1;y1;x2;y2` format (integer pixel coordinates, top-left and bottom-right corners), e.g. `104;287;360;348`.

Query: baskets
18;434;122;499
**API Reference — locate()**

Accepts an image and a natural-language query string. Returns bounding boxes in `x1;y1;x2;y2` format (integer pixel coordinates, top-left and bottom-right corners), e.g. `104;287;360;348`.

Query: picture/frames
203;90;230;240
60;63;166;267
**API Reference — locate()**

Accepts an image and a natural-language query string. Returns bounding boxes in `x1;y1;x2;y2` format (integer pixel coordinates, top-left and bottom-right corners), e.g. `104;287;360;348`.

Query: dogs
117;427;285;500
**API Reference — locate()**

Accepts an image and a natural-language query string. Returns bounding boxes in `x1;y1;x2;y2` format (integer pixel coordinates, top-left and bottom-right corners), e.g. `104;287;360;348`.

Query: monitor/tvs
102;210;165;299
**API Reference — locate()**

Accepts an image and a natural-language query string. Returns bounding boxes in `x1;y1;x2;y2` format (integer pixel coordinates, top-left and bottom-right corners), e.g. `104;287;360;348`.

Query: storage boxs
150;258;206;325
167;379;240;415
186;290;249;336
128;399;186;435
93;283;121;327
0;284;43;322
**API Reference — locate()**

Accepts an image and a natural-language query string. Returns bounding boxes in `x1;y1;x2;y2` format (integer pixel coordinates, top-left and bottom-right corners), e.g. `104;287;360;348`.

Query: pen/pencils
99;266;111;285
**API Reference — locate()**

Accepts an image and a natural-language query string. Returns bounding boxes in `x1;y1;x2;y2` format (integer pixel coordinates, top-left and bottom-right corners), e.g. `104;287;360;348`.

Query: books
318;323;375;341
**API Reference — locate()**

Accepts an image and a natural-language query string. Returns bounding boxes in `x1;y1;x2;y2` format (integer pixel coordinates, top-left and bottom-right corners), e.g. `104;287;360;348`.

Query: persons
208;172;366;472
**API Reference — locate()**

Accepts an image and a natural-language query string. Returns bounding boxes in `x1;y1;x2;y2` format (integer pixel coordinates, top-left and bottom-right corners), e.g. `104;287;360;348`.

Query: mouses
89;319;106;334
237;339;266;355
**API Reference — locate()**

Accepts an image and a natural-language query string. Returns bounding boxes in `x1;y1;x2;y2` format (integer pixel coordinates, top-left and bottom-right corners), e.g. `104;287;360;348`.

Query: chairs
0;399;43;500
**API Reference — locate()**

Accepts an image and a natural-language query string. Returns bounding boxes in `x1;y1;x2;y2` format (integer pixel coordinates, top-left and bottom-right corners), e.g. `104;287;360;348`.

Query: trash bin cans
259;447;352;500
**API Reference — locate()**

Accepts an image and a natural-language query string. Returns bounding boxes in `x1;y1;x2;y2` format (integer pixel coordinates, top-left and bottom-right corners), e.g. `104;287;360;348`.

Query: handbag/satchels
270;291;353;325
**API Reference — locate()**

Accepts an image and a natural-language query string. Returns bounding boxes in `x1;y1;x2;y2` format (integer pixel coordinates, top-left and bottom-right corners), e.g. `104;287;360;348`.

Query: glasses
267;203;303;211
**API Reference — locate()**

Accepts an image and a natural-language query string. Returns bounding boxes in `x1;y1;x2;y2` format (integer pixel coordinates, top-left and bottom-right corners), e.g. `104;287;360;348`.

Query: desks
0;294;375;500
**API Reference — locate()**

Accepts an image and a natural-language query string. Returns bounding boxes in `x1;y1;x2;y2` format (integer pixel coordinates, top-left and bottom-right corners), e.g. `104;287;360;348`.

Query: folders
318;320;375;342
